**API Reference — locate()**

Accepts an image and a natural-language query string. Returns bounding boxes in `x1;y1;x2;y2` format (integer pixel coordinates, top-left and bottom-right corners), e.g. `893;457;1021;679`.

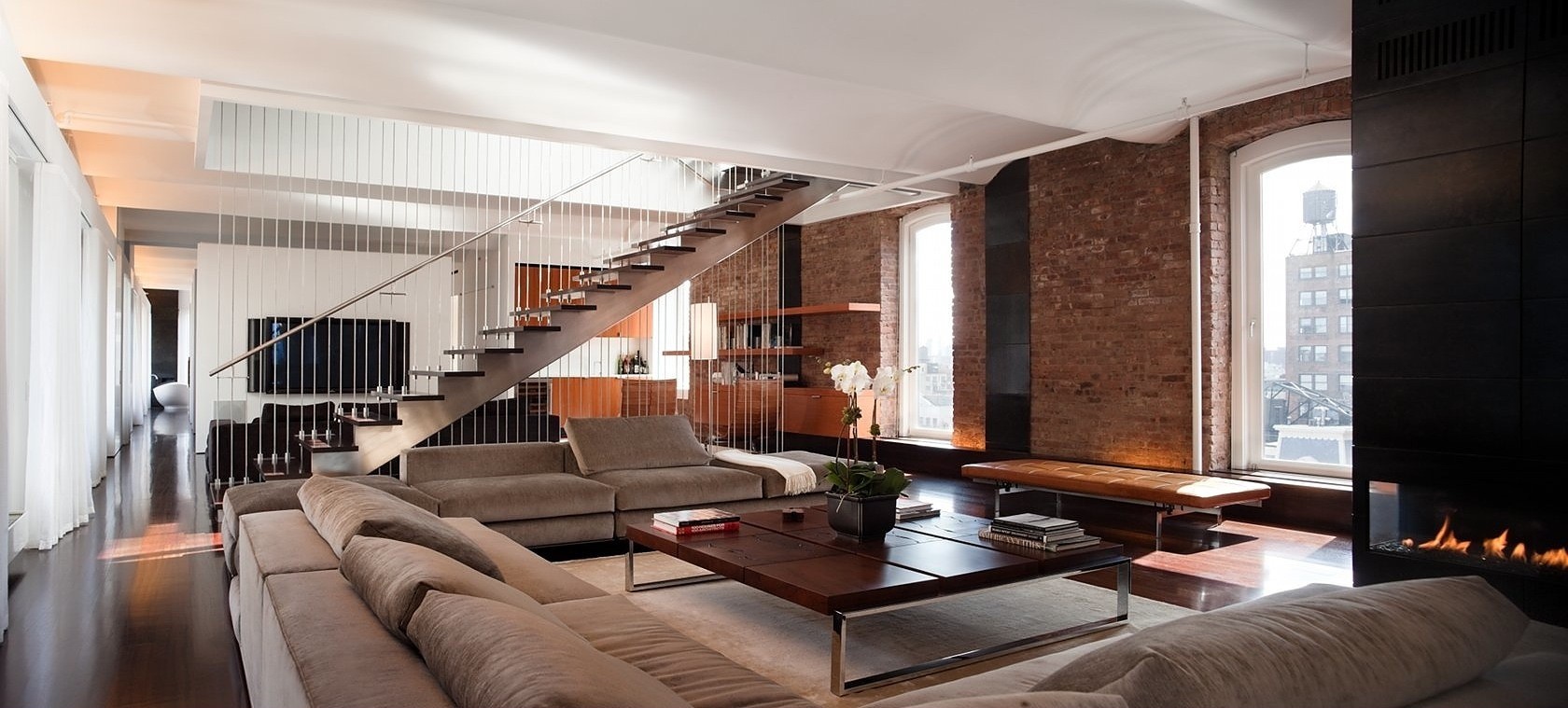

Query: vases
826;490;897;546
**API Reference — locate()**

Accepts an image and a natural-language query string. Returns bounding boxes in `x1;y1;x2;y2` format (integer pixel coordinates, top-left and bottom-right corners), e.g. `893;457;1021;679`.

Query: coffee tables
623;503;1136;696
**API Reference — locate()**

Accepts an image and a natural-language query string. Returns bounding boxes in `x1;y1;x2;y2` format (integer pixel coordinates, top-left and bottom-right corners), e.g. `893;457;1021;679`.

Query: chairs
153;383;191;414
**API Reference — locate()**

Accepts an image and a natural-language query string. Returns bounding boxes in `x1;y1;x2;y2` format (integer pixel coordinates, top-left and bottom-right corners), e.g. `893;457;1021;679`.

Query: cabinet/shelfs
691;303;880;438
553;377;678;424
515;378;552;418
516;261;655;336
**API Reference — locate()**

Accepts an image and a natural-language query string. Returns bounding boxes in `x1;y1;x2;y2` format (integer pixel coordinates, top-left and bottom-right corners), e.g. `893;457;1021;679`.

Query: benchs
963;457;1271;558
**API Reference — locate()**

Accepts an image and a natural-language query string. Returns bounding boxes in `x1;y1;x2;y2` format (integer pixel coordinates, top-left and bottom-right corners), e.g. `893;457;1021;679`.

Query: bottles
623;356;631;374
643;360;649;374
631;350;643;374
618;355;623;374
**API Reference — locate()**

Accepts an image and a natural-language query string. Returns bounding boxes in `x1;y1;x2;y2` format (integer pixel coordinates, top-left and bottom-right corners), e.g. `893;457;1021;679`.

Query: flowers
809;349;923;498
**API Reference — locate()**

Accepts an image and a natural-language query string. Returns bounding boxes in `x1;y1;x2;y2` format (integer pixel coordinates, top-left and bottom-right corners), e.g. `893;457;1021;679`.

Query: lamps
691;303;718;443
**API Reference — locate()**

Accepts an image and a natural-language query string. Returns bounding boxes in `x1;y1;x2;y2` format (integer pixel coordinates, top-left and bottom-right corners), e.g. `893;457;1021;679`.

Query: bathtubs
152;382;189;409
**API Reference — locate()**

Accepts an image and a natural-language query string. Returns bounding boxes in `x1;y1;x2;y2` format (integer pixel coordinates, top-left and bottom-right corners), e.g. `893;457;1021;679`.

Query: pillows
338;534;591;651
296;473;508;583
563;414;711;477
262;400;335;426
405;591;693;708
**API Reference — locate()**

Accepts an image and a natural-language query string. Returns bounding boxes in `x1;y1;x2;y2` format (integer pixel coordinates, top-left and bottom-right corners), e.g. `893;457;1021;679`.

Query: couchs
218;411;1568;707
205;398;561;489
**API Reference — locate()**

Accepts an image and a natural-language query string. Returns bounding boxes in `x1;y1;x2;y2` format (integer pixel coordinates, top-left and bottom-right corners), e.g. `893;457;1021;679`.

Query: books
978;513;1100;552
895;498;941;520
719;323;777;350
650;507;740;536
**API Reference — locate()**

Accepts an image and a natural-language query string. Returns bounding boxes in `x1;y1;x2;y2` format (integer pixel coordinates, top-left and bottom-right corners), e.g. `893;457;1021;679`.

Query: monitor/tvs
248;318;410;394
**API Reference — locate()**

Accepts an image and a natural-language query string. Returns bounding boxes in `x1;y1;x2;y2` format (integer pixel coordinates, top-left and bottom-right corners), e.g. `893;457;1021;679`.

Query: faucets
151;373;158;381
